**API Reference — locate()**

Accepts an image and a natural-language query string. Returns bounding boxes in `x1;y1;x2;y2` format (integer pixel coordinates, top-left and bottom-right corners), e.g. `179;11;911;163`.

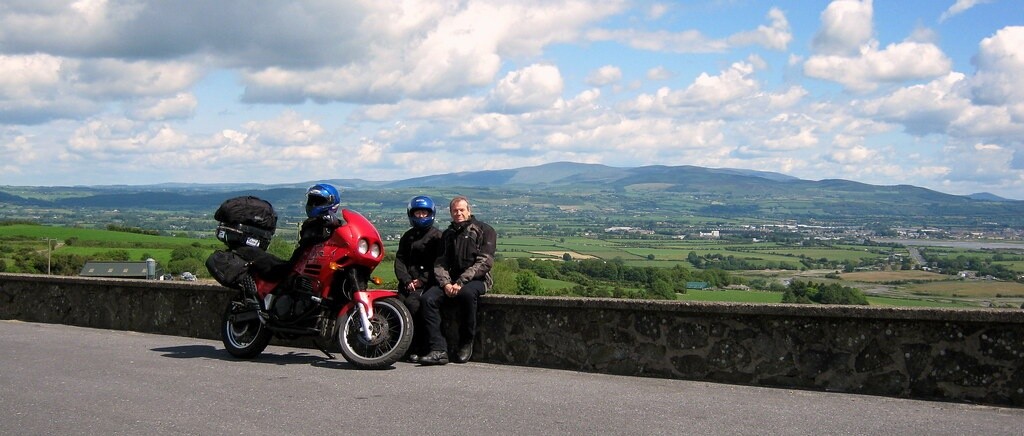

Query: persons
391;196;442;363
421;197;497;365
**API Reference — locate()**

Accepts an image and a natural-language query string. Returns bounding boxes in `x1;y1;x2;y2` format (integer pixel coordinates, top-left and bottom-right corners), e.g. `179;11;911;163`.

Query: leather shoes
455;344;472;362
418;350;449;365
408;354;419;362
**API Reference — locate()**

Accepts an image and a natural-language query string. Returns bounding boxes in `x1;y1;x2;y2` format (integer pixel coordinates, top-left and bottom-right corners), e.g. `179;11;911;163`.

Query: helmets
407;196;436;230
305;183;340;217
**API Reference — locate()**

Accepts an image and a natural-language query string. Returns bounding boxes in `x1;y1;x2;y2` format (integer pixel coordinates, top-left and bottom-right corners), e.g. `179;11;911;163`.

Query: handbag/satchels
206;249;254;287
214;196;277;231
298;216;334;247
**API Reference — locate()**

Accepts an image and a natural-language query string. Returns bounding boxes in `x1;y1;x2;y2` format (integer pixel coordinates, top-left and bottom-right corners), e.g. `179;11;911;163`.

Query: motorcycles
214;211;415;369
